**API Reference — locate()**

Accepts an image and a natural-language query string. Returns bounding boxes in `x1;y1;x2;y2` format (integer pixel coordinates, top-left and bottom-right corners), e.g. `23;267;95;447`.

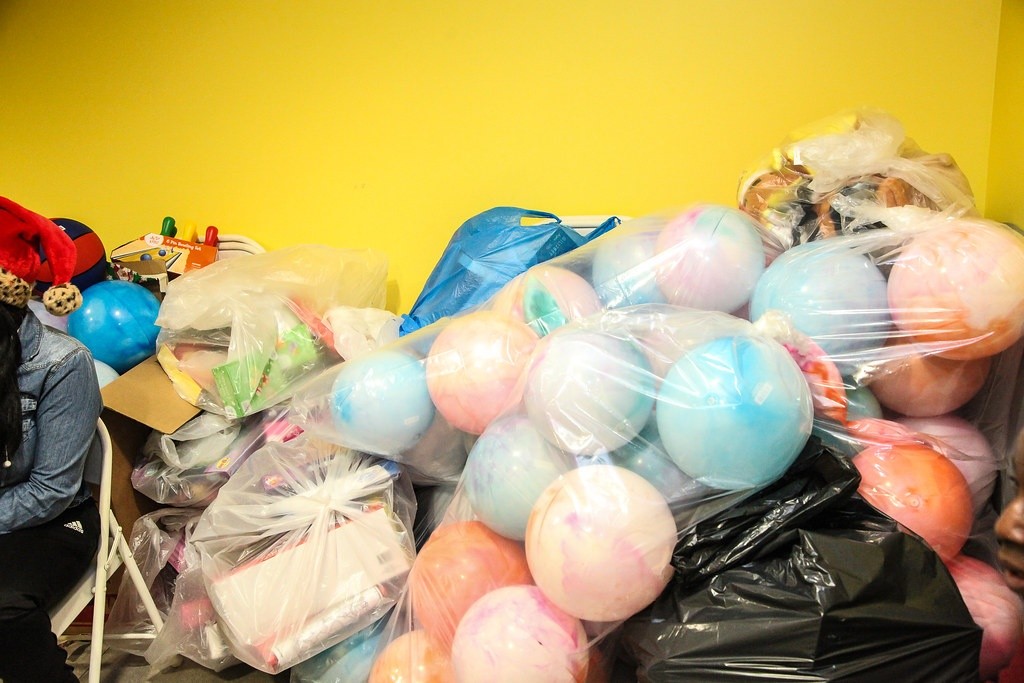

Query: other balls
66;281;163;372
329;206;1024;681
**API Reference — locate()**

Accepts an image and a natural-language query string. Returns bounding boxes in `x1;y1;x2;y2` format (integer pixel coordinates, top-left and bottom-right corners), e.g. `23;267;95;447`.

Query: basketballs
32;217;106;297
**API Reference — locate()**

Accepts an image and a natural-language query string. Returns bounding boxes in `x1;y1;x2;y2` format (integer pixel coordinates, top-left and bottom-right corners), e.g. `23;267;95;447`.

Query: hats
0;196;83;316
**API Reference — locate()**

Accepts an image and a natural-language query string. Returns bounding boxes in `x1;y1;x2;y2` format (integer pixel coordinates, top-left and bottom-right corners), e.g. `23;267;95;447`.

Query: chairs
46;417;180;682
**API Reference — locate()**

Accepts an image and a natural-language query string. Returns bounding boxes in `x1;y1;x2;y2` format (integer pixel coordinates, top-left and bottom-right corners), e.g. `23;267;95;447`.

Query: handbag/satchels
400;208;621;336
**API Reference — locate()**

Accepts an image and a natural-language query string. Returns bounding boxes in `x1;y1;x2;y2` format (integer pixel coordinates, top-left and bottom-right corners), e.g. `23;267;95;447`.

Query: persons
0;198;104;683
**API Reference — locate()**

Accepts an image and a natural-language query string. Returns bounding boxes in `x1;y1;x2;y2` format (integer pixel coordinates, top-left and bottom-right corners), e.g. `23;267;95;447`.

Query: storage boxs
100;339;204;596
